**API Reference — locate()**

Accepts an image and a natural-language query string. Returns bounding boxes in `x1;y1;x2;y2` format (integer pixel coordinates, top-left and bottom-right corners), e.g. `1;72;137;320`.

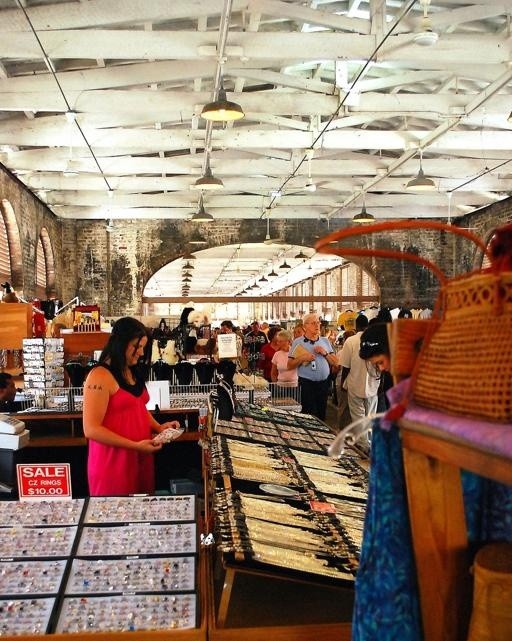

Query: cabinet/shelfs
0;495;208;641
0;386;210;446
206;387;374;641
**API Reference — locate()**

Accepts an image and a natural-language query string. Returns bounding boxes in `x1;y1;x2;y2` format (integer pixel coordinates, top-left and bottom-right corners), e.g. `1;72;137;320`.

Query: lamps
416;0;439;46
404;168;435;192
234;283;259;297
304;149;317;193
195;175;225;191
191;207;213;222
278;261;292;269
353;208;374;223
200;89;245;122
296;251;309;259
268;269;278;276
181;254;197;297
188;232;206;245
258;275;268;281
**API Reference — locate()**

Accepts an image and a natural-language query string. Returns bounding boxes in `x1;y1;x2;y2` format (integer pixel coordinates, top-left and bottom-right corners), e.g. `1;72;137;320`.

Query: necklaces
304;467;367;499
240;494;352;580
325;496;366;569
228;439;301;487
293;448;351;476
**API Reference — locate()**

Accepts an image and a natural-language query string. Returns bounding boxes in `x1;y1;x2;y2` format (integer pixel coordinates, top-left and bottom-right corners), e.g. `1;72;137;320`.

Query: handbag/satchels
316;221;511;422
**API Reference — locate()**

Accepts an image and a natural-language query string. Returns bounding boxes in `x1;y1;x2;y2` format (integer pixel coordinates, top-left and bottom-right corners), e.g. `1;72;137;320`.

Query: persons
287;315;337;421
0;373;15;409
83;318;180;496
221;321;242;373
358;324;393;416
293;325;305;342
340;316;380;457
258;326;282;383
244;323;269;370
272;331;298;399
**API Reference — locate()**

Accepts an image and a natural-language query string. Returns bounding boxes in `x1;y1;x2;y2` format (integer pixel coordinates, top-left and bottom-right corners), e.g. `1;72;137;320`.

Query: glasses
305;321;320;325
